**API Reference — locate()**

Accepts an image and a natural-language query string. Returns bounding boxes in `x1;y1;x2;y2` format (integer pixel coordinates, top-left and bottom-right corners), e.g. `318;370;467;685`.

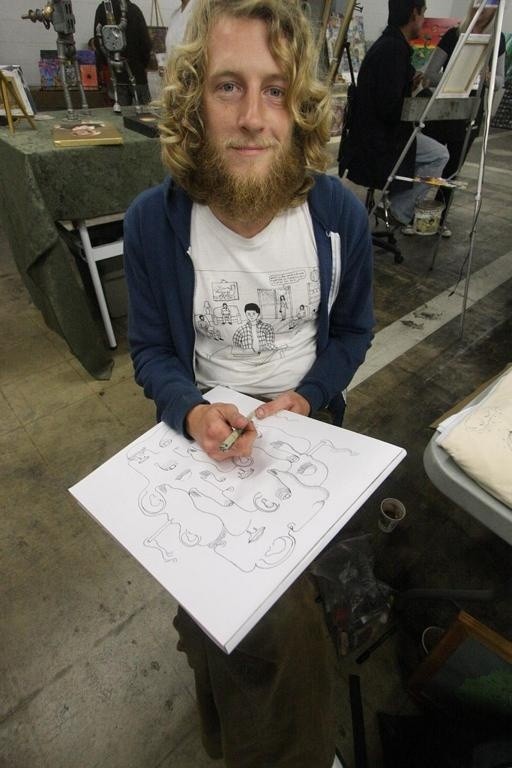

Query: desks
1;100;171;351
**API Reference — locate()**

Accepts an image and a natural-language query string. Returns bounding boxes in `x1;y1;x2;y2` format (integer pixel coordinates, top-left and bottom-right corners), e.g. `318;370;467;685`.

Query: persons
422;0;506;237
123;0;375;461
339;0;450;228
94;0;151;106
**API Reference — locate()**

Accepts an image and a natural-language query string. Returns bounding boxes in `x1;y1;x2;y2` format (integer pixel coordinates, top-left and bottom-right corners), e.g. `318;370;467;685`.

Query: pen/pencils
220;411;255;452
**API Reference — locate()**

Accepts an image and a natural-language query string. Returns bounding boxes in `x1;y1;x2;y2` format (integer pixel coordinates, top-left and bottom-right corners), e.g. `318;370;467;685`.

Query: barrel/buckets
414;201;446;236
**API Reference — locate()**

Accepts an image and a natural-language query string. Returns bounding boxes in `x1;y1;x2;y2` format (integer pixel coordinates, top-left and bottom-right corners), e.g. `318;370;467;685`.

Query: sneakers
373;205;405;226
438;225;451;238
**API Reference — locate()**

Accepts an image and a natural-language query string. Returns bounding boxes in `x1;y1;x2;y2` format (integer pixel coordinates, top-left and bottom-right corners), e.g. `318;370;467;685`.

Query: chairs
338;82;406;265
